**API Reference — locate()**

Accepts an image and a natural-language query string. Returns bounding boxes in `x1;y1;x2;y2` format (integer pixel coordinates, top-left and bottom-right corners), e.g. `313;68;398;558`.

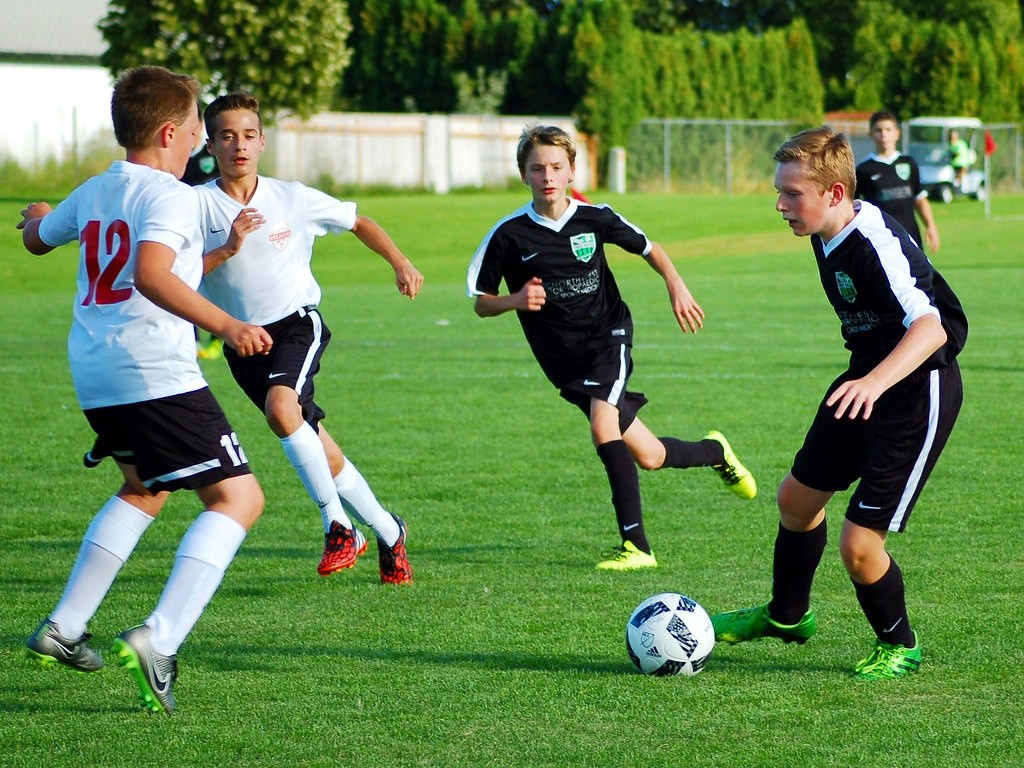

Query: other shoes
198;336;222;361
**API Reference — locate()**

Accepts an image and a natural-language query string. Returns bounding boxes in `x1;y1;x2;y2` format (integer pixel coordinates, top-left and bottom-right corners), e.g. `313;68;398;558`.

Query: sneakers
703;429;756;500
851;631;920;682
594;541;658;571
112;624;176;716
378;512;410;586
710;601;815;647
26;620;103;674
318;520;367;576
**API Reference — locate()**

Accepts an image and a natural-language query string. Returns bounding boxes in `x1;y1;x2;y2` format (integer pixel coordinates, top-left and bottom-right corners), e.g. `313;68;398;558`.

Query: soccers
625;591;716;677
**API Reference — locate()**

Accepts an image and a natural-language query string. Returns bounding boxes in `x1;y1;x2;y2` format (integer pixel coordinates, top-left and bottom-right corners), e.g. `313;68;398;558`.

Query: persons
466;125;758;572
712;127;969;682
853;111;939;257
16;66;274;720
940;128;968;188
181;93;423;583
